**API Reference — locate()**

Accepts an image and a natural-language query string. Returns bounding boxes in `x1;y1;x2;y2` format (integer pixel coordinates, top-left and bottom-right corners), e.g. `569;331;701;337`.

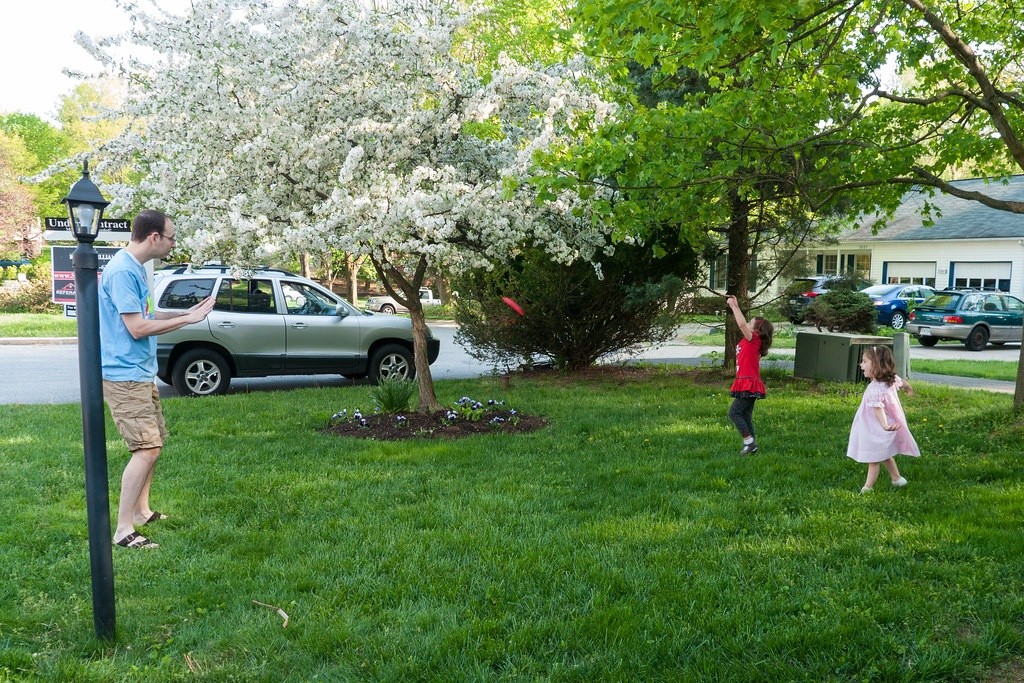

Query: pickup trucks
365;286;442;315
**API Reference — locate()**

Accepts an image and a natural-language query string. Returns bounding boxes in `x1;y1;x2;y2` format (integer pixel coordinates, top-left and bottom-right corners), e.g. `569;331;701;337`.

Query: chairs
250;289;273;312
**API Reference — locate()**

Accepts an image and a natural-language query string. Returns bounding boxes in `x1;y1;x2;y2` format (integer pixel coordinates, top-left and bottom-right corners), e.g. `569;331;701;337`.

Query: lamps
59;159;109;246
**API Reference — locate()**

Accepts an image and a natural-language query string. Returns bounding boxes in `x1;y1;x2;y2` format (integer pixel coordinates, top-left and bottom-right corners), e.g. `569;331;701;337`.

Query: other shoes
740;442;758;455
892;477;908;487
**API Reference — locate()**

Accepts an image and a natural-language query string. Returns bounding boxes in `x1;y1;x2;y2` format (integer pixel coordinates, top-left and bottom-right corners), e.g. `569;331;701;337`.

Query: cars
858;284;938;330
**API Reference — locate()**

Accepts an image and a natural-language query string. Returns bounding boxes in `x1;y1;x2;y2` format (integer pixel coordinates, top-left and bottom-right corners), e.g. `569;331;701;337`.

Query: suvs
776;274;876;325
904;286;1024;351
149;263;442;398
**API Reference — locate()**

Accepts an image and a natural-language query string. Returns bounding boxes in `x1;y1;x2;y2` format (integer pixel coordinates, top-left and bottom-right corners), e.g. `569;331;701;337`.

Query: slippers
117;531;152;550
146;511;161;523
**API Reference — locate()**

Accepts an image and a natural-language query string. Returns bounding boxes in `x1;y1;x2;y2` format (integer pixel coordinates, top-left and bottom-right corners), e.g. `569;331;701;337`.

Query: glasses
144;233;177;243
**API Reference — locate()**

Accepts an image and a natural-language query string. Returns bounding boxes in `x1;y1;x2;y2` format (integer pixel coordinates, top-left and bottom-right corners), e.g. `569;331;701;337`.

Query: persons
97;209;217;550
726;295;774;456
846;344;921;495
